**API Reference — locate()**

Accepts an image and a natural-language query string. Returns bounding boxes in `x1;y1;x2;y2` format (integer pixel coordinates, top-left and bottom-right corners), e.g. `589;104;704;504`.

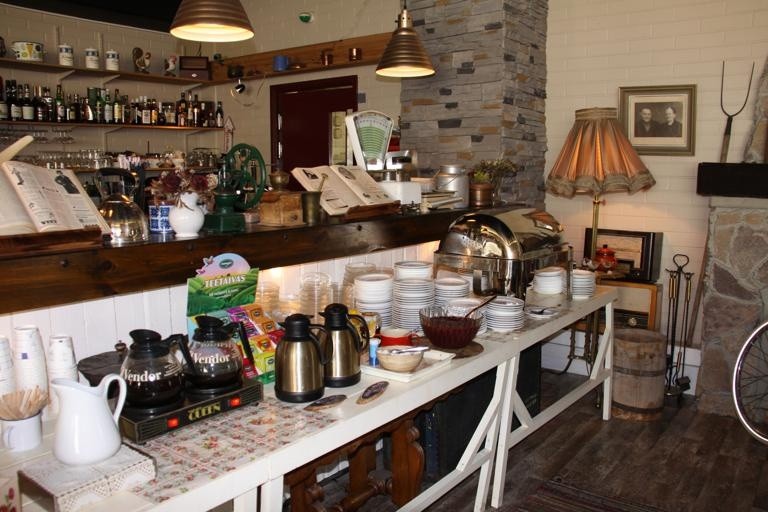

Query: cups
35;149;113;170
147;205;173;234
254;263;375;322
227;64;243;78
349;47;361;61
0;412;43;453
48;371;128;466
320;55;332;66
380;328;420;348
273;55;289;72
0;324;79;419
301;191;322;225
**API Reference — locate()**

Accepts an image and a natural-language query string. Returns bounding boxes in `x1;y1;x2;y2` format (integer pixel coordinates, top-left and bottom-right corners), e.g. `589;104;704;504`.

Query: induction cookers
76;344;264;445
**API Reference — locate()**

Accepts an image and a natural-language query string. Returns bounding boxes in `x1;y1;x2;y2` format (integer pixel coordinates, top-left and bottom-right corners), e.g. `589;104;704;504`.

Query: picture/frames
614;81;699;160
582;227;657;284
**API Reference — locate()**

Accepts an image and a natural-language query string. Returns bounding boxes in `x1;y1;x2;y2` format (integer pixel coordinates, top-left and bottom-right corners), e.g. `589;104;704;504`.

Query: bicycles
732;315;767;445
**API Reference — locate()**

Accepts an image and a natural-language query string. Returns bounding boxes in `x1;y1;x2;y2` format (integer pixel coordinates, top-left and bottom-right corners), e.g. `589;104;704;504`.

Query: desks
0;282;624;512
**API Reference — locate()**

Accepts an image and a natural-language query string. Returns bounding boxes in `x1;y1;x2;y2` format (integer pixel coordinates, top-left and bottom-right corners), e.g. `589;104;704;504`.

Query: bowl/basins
377;345;425;373
410;177;436;193
11;41;43;62
419;303;484;349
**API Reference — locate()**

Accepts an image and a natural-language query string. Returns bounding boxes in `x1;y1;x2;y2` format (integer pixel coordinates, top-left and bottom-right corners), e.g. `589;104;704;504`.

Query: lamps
168;1;256;44
534;106;660;416
373;1;438;80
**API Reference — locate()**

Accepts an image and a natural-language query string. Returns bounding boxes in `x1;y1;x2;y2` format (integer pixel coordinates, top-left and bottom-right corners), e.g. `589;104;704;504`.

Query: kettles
272;313;333;403
314;302;370;388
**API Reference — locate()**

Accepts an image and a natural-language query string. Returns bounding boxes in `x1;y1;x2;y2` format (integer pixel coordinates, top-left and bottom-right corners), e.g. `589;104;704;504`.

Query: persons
657;105;682;138
635;106;661;137
54;171;81;194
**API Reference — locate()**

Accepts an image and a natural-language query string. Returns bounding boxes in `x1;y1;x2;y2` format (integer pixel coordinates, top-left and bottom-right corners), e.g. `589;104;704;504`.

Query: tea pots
92;168;152;244
183;314;255;403
121;328;199;416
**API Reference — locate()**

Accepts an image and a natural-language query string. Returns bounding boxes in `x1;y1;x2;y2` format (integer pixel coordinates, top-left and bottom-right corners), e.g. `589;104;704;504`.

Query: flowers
147;165;221;216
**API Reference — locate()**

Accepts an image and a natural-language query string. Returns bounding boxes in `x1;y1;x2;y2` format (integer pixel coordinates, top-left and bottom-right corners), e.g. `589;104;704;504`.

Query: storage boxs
176;55;228;84
256;191;305;230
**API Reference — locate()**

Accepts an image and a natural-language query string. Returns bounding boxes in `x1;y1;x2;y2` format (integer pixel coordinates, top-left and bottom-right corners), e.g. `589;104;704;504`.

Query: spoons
531;306;557;314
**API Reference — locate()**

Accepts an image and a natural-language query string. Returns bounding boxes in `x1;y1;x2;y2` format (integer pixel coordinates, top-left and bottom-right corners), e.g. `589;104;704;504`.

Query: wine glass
0;126;76;145
268;171;291;194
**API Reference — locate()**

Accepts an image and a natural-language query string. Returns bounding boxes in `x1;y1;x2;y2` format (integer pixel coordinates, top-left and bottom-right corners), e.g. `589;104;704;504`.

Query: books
289;163;397;217
0;160;114;239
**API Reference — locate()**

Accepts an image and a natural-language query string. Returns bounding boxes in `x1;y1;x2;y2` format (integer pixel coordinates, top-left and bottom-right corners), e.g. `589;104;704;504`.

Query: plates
354;260;487;335
532;266;596;300
524;307;560;318
480;295;527;334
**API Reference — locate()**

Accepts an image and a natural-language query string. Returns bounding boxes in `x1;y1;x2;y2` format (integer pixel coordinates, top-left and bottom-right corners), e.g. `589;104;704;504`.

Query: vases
167;191;207;240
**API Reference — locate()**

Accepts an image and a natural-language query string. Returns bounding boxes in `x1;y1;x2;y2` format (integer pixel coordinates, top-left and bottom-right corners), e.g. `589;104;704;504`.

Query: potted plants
466;170;499;210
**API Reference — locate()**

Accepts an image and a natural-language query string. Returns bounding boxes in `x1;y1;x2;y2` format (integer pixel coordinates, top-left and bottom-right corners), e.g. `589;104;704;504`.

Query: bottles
85;49;100;70
348;310;381;367
144;153;161;169
595;244;616;272
0;76;224;128
58;45;73;67
208;151;214;167
105;51;120;71
438;166;468;207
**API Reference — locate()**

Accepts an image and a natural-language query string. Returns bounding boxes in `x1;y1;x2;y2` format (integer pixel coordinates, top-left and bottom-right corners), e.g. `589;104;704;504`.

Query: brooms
674;273;692;386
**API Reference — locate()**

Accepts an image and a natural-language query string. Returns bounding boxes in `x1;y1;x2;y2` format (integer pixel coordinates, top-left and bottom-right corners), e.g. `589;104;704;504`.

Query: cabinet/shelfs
0;57;226;180
